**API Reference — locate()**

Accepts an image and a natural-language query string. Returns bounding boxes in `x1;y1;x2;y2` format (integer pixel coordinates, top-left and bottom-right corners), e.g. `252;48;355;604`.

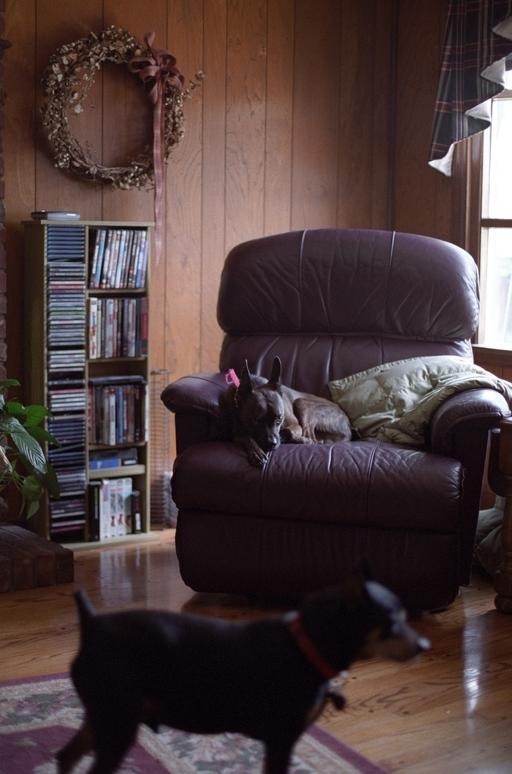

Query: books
45;225;150;550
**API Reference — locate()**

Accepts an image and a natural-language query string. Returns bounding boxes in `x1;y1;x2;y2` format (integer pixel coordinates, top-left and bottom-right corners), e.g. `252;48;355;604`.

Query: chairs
163;228;511;616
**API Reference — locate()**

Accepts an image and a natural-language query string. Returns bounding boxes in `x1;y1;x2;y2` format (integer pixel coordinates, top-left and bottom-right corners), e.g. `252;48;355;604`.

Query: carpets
1;672;384;774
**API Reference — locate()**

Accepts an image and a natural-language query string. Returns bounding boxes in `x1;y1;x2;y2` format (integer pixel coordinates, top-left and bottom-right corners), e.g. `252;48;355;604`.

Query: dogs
51;554;434;774
223;354;353;469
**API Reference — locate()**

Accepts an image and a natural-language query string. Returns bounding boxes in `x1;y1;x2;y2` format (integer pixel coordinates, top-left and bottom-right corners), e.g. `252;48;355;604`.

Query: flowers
38;21;203;194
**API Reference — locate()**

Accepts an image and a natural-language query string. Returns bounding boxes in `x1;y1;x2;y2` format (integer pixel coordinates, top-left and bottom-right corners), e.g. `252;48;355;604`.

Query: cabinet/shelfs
19;218;155;550
